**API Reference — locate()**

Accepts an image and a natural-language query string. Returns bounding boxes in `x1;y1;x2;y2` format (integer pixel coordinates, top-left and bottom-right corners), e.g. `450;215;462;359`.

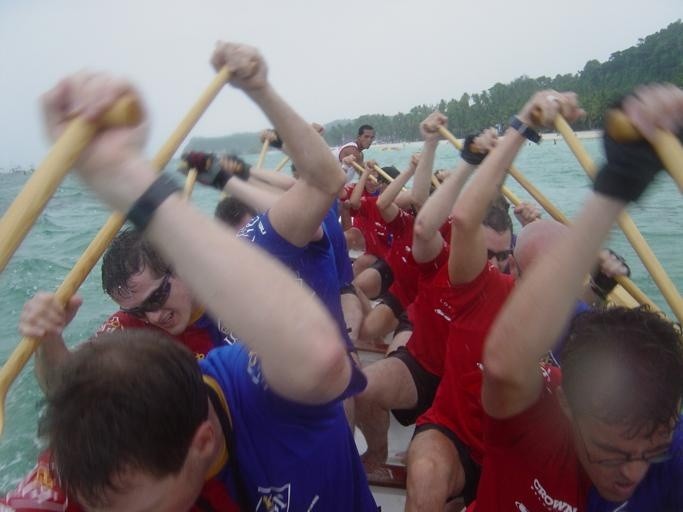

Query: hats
377;166;400;186
215;197;248;226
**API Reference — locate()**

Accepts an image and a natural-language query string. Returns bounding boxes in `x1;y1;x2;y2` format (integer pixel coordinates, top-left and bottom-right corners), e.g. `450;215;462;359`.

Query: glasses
488;248;513;262
578;426;675;466
119;272;171;318
